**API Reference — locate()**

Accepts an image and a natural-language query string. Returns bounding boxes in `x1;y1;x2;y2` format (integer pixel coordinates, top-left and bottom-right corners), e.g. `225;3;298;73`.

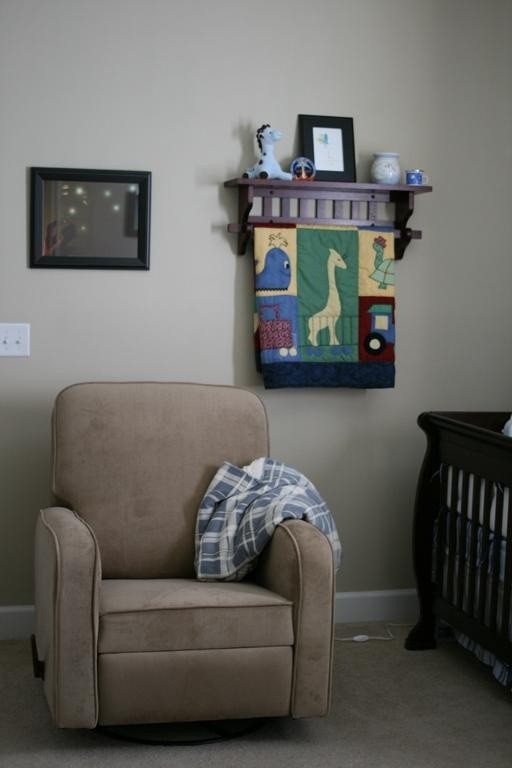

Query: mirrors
28;167;152;270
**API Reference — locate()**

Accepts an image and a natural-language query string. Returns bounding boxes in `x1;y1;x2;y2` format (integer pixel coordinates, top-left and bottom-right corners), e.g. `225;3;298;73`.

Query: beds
401;405;510;700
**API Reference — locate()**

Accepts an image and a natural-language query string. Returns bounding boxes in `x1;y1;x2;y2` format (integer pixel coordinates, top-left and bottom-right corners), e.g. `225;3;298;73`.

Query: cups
406;170;430;186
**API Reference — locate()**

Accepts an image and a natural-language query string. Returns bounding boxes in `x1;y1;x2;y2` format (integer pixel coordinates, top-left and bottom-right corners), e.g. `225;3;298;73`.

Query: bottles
370;153;400;184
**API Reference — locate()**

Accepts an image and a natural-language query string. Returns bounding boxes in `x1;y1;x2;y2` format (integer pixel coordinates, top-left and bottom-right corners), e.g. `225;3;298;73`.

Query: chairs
29;381;337;746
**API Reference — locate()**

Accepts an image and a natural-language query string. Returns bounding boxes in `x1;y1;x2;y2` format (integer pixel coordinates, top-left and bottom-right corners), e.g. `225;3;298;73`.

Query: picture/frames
298;113;356;183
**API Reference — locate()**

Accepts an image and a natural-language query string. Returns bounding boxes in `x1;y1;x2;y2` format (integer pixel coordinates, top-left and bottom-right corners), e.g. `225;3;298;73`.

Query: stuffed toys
243;124;294;183
